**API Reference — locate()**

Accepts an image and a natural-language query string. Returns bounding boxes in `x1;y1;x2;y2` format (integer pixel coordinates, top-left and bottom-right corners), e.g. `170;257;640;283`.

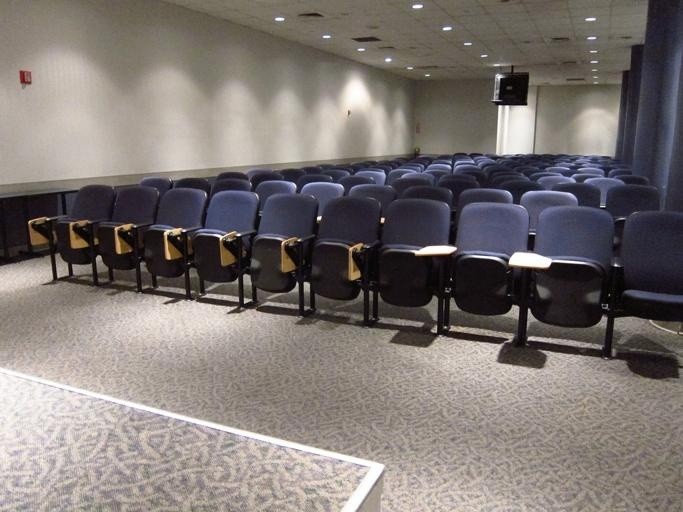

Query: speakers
491;72;529;106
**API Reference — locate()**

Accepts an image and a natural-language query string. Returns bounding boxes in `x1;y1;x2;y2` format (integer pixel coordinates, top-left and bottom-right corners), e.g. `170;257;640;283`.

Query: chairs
530;172;560;180
217;171;249;182
33;185;116;284
498;180;546;203
296;193;381;326
537;176;576;190
546;166;570;175
87;184;160;295
554;181;600;207
453;187;514;246
494;175;529;187
615;175;649;185
518;190;580;252
400;185;453;207
138;176;173;197
251;174;284;190
355;171;386;187
610;209;683;359
300;181;345;225
513;205;613;360
301;166;323;175
571;173;603;182
317;152;636;171
585;176;626;203
254;180;297;219
608;169;633;178
130;188;208;298
576;167;606;177
454;165;481;181
489;171;521;183
203;178;253;199
390;178;432;196
321;169;350;183
441;201;529;342
348;184;396;206
280;168;307;182
427;164;452;175
386;168;416;184
513;166;540;177
235;191;319;315
181;189;259;308
363;197;452;333
604;184;660;268
437;173;481;207
173;177;211;198
424;169;450;182
338;176;376;196
297;174;333;192
402;172;437;185
